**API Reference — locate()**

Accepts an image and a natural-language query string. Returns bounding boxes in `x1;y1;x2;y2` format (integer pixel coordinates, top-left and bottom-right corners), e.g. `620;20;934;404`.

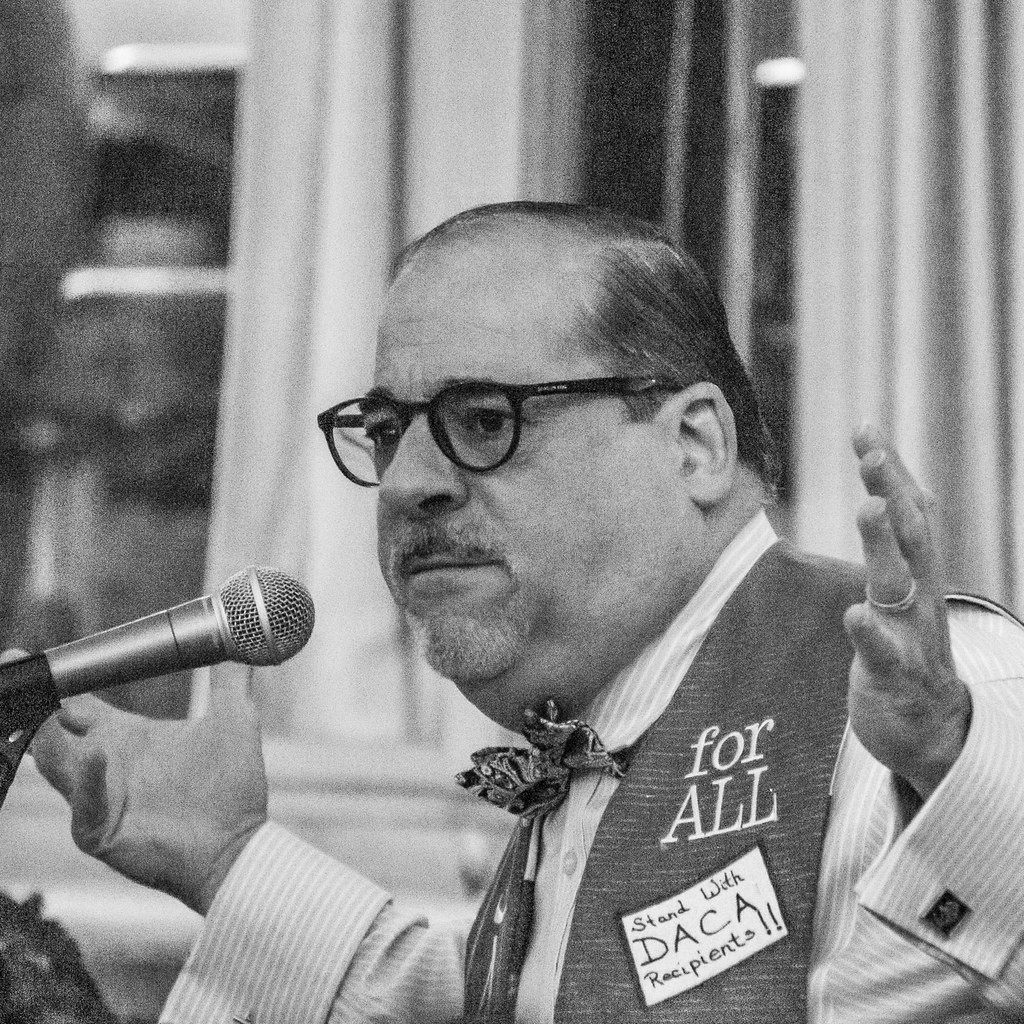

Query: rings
865;576;916;613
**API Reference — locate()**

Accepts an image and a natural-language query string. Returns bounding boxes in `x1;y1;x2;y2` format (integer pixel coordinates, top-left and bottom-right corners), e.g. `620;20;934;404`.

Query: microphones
0;567;317;713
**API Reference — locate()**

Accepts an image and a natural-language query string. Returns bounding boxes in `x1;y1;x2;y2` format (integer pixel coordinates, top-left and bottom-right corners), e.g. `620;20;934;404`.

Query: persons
1;201;1024;1024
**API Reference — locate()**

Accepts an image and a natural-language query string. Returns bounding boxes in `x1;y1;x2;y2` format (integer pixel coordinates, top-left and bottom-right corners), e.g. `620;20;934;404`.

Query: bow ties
455;699;635;817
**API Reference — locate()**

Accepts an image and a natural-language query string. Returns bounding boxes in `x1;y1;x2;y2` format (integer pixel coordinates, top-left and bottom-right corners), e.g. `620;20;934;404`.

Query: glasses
317;377;692;490
